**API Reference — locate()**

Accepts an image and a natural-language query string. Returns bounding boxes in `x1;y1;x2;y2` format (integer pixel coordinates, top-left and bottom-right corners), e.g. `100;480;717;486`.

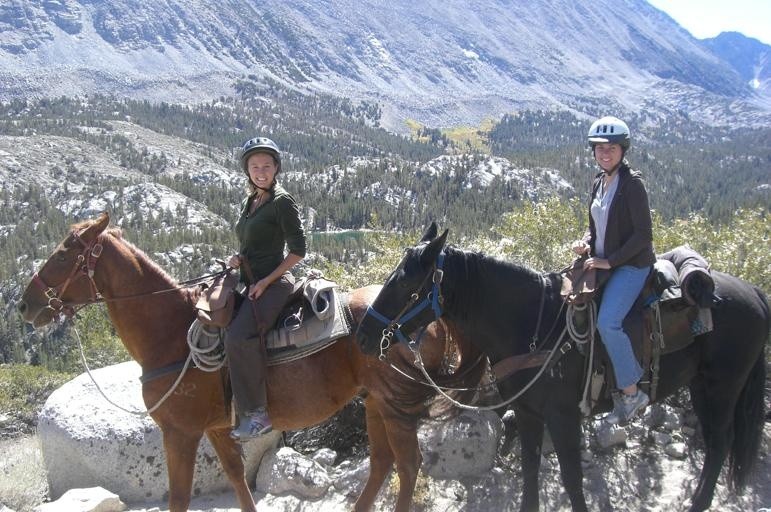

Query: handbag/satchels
560;267;598;305
196;285;236;328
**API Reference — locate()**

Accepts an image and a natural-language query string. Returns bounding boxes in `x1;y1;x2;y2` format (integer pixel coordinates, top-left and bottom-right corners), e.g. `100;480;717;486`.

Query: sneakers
229;406;273;440
605;388;649;424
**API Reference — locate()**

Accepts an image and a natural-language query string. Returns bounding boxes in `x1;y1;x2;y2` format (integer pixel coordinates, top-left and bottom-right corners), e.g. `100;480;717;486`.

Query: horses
355;220;771;512
17;211;489;512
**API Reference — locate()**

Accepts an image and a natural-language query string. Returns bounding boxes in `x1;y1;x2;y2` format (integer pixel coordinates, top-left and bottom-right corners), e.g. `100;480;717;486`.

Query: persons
221;135;310;444
571;114;655;426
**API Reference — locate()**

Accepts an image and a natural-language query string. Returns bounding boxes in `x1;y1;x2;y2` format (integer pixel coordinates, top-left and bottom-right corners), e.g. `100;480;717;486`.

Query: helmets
241;136;281;177
587;116;630;151
